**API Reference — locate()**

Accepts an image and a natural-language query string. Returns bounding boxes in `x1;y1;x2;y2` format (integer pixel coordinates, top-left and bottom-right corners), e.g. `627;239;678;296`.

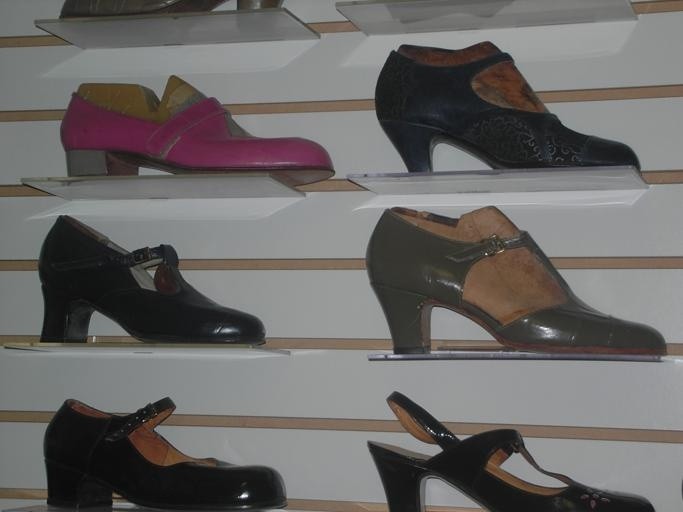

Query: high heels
367;391;655;511
38;215;267;344
44;396;287;512
60;91;335;186
375;50;641;170
365;207;668;356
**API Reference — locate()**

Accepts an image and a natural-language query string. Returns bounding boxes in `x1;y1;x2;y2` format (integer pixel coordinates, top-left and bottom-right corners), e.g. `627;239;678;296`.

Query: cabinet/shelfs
0;0;667;361
